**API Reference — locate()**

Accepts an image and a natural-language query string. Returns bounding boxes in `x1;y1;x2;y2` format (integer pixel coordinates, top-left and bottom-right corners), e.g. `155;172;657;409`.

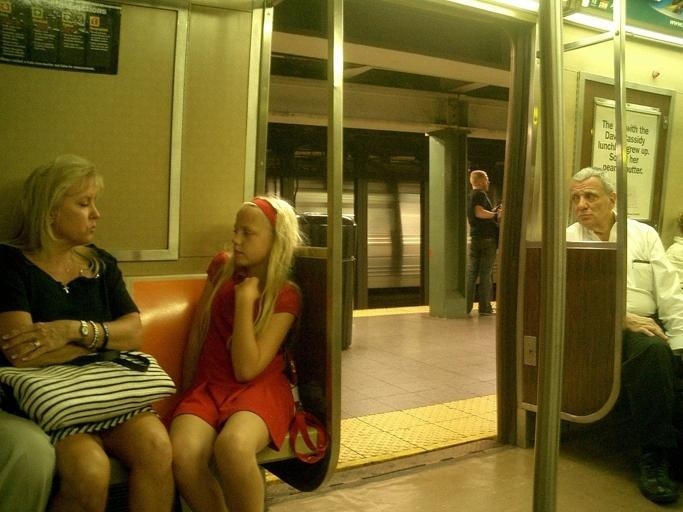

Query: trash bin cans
299;212;356;351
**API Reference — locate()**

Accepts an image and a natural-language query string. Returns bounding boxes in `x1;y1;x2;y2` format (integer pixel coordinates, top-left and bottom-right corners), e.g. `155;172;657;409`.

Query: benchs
106;272;327;512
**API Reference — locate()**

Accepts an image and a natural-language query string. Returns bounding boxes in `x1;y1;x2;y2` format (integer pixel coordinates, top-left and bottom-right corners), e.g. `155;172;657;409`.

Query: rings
34;341;40;348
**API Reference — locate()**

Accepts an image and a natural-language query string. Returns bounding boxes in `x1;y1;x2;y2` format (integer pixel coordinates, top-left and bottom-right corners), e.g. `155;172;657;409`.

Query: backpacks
283;327;327;425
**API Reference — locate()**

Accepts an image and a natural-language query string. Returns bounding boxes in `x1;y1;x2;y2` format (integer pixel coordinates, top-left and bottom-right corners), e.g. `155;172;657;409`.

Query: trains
267;175;500;289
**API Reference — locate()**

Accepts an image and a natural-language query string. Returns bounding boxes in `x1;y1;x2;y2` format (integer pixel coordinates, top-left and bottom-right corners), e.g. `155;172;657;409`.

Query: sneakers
466;304;496;314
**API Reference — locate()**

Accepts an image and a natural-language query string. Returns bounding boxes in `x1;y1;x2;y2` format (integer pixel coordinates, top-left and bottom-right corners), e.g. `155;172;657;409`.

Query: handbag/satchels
0;350;177;433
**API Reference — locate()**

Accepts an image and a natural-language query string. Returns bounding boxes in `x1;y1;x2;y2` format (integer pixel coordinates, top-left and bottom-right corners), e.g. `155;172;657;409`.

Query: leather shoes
638;457;679;504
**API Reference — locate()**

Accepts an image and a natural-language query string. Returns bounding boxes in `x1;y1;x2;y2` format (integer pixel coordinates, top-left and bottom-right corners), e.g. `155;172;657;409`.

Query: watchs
79;320;89;342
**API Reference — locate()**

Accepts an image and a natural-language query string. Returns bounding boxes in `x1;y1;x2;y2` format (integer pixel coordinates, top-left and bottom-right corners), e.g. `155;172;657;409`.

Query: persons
0;152;173;512
566;167;683;504
466;170;502;315
170;194;308;512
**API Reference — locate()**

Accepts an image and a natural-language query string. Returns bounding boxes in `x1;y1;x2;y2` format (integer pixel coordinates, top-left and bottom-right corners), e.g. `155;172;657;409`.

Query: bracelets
89;321;99;348
100;321;109;350
